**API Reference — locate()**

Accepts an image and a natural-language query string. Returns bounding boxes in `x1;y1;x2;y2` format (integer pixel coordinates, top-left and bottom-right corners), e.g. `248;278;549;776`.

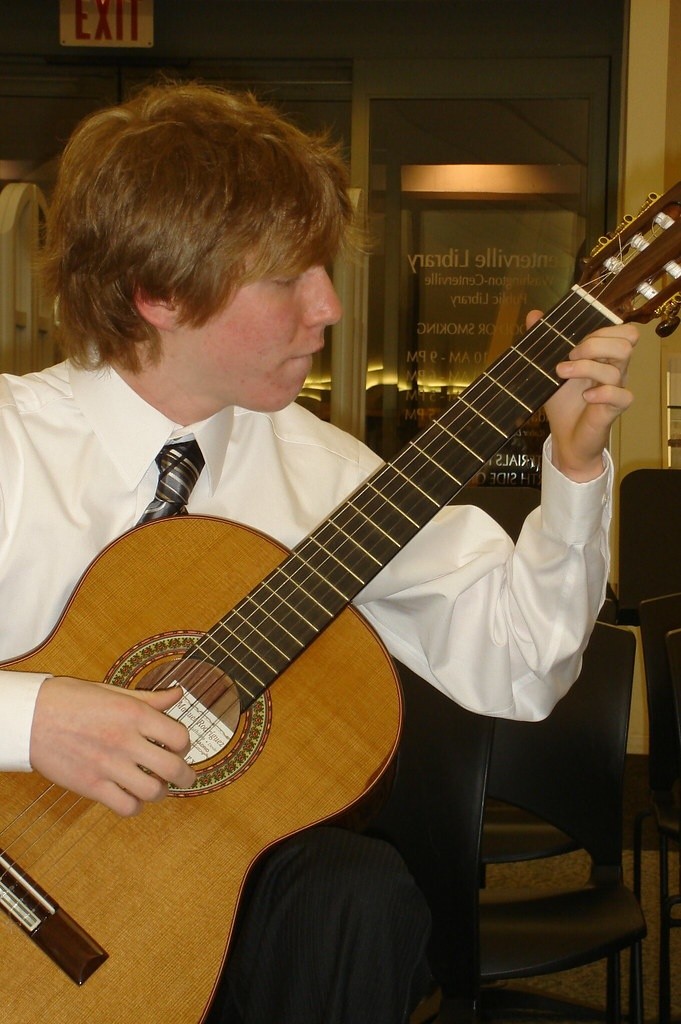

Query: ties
134;440;206;527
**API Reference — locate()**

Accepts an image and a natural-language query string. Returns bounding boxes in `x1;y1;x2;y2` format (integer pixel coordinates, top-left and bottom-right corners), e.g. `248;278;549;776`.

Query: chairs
205;468;681;1024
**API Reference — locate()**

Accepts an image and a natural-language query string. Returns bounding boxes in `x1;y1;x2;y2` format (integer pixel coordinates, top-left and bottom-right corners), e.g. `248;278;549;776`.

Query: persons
0;84;639;1024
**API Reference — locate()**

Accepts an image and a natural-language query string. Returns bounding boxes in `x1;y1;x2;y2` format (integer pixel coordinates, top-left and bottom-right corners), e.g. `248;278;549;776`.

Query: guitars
2;180;681;1023
